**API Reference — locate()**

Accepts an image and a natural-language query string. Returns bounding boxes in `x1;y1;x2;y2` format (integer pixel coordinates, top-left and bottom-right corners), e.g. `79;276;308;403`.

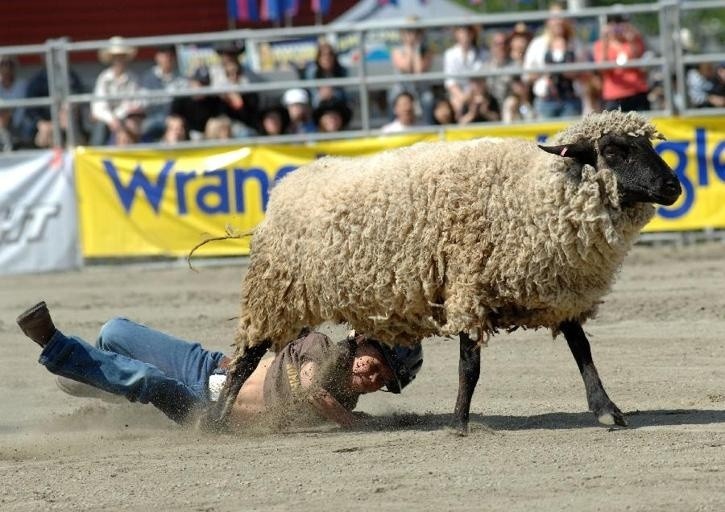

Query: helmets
377;340;423;394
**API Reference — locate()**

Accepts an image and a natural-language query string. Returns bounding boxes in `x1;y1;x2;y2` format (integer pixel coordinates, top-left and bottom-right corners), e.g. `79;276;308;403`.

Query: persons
380;4;724;132
1;37;356;150
15;301;424;435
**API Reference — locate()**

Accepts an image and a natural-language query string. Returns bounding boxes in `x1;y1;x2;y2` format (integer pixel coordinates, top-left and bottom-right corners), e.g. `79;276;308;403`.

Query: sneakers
56;375;126;405
17;301;54;342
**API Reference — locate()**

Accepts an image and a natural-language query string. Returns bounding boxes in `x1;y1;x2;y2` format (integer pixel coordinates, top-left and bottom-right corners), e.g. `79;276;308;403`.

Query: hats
98;36;135;61
284;89;309;105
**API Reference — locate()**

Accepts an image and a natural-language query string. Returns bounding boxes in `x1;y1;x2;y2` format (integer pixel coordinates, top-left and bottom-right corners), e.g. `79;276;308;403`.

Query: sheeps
198;108;683;440
